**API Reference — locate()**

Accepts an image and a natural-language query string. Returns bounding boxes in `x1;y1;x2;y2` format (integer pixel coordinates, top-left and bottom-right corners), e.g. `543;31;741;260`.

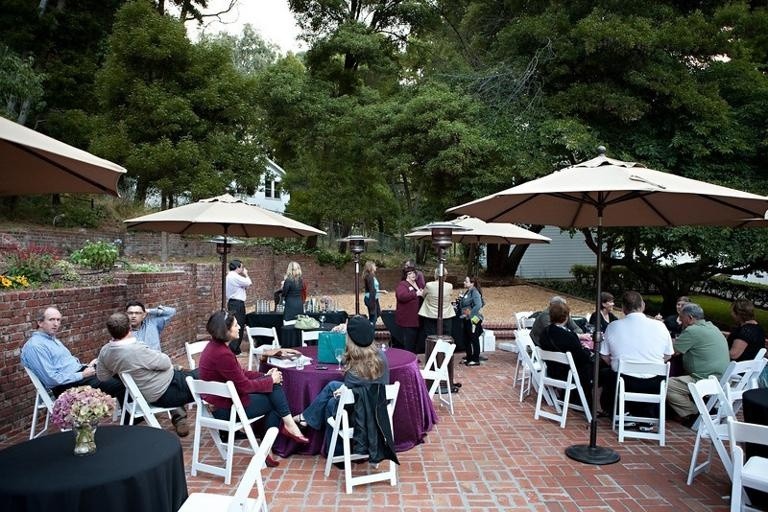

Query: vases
74;430;98;455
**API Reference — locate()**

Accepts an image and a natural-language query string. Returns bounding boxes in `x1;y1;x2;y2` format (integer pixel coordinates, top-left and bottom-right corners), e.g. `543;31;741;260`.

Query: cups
296;358;304;370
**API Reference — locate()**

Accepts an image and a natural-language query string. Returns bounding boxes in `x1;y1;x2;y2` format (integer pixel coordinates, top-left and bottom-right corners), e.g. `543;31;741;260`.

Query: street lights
201;234;245;315
335;234;377;316
411;223;473;392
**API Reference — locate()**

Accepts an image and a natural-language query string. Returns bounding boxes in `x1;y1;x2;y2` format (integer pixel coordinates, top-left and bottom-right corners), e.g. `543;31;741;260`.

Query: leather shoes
177;424;189;437
466;361;480;365
265;454;279;467
280;421;310;444
460;359;467;364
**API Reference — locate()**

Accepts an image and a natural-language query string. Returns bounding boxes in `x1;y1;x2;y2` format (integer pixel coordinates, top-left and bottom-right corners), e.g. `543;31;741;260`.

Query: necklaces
600;311;610;323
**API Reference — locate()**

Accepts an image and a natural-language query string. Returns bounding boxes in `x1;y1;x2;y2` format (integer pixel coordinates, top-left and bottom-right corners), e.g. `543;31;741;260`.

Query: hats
348;315;375;347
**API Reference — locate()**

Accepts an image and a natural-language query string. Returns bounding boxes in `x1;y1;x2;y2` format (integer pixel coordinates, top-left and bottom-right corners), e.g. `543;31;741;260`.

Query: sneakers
683;414;698;426
638;422;653;431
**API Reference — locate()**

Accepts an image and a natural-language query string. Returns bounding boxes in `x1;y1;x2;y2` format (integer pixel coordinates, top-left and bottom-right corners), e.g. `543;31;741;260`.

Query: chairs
25;366;73;439
120;373;178;430
246;326;281;371
283;319;297;326
179;426;279;512
420;338;456;415
324;380;400;494
186;376;267;485
185;341;209;409
301;330;320;346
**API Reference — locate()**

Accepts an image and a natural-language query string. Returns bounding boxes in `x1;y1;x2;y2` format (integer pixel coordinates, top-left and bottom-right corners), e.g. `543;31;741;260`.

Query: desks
281;322;339;348
245;311;349;348
381;309;467;353
0;426;188;512
260;347;438;457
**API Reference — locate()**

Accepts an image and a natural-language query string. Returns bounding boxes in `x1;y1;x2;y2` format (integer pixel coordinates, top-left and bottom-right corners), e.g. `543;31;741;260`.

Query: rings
279;372;281;375
280;375;282;379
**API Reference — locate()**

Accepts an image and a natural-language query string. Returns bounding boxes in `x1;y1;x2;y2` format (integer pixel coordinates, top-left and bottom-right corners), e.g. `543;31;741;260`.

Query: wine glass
388;304;392;313
335;349;344;370
319;315;326;328
379;341;389;352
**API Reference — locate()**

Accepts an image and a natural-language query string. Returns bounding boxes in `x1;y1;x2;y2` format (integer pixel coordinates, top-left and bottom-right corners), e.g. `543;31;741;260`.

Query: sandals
596;408;608;418
295;413;308;434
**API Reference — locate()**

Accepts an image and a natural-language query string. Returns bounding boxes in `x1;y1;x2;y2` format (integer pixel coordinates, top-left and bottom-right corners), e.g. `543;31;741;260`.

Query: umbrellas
444;152;768;447
0;116;127;199
404;215;552;274
123;190;327;312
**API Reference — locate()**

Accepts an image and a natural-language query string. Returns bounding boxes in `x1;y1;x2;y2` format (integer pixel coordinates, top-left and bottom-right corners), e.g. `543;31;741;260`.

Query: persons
225;259;253;355
96;312;248;442
126;301;189;436
198;311;310;466
528;296;584;347
418;266;456;335
405;259;425;289
589;292;619;333
362;261;389;328
665;296;692;338
292;315;390;470
599;292;675;431
395;266;424;364
281;261;306;321
20;306;145;425
722;301;766;377
539;302;613;418
459;274;486;366
666;303;730;427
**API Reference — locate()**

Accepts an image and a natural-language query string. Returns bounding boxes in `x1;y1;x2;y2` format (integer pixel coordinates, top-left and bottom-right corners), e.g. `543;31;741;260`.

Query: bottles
600;332;605;341
305;295;320;314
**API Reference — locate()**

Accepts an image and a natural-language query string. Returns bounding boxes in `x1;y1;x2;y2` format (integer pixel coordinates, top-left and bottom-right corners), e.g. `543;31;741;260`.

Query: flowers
52;386;118;429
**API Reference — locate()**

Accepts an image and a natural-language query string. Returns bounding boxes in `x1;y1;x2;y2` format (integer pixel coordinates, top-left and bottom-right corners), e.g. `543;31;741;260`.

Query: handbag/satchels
294;314;320;330
317;331;346;364
262;348;302;361
471;298;485;308
